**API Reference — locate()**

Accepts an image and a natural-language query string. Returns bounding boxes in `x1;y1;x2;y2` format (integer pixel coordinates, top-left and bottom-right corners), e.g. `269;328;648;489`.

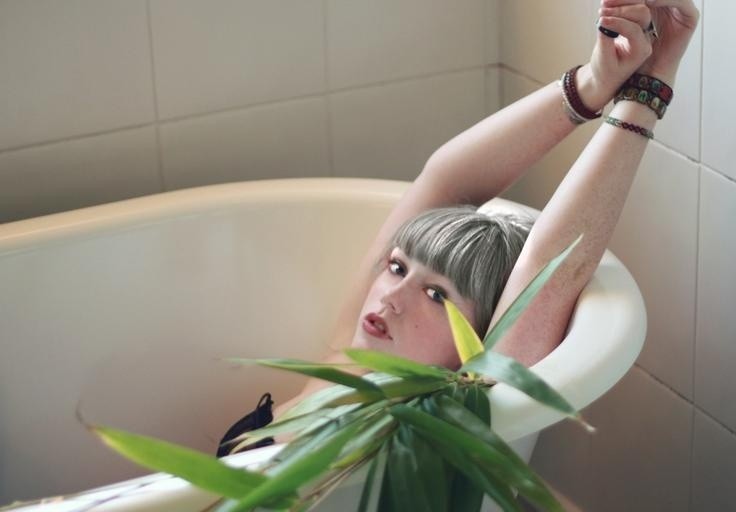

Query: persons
208;0;699;457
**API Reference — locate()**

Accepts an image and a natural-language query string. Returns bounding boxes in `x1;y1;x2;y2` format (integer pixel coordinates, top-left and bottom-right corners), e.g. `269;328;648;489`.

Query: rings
644;19;659;42
597;21;619;38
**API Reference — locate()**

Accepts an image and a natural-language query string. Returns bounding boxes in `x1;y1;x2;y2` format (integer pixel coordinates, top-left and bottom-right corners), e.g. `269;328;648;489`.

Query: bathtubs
0;176;648;511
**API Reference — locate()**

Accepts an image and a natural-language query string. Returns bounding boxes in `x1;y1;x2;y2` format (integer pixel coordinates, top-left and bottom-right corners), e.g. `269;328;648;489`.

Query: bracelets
558;74;591;124
612;87;670;118
561;65;605;122
623;71;674;102
607;112;656;142
